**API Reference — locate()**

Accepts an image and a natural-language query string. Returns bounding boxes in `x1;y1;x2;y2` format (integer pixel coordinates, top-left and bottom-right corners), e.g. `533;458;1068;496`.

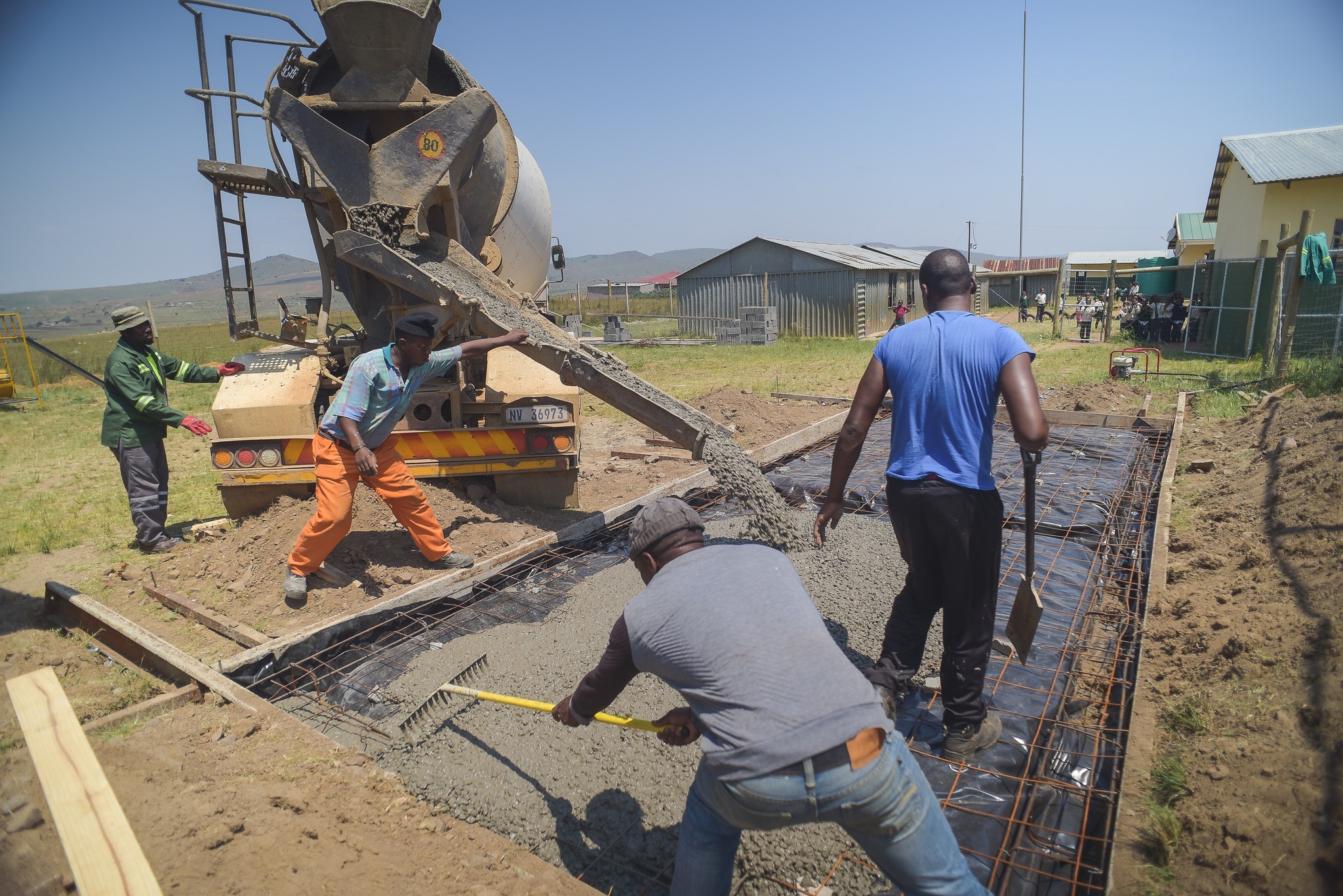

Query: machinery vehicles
179;2;736;516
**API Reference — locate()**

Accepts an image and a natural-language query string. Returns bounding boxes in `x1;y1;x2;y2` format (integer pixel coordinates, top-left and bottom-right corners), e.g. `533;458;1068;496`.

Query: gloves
180;415;212;437
217;361;245;376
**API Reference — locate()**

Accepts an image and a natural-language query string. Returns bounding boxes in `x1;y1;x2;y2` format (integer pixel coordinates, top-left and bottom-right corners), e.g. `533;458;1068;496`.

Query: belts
774;742;850;776
926;473;939;480
318;427;377;452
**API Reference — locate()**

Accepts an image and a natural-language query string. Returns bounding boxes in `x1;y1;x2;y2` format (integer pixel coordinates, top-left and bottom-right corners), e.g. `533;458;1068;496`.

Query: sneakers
282;566;308;600
943;711;1002;761
139;537;184;554
427;552;474;568
871;683;898;729
166;534;184;541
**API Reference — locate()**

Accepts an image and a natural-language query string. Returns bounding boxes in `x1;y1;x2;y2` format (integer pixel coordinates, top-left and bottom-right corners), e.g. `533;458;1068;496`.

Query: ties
1128;303;1134;314
1155;304;1159;318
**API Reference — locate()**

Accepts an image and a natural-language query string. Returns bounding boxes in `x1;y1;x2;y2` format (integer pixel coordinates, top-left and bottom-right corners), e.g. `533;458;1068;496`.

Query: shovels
1005;446;1044;667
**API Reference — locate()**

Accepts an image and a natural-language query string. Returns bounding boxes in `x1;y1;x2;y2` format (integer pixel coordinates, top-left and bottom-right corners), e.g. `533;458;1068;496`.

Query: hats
628;497;705;560
394;311;439;338
110;305;152;331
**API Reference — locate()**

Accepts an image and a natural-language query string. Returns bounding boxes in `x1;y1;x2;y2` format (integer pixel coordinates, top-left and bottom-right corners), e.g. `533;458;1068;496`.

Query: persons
1080;298;1098;343
814;248;1048;762
1092;295;1106;330
1019;291;1030;323
1076;295;1084;327
1035;288;1046;323
1092;287;1098;303
887;300;911;333
1059;293;1070;319
1104;286;1110;303
1129;281;1139;298
1116;291;1212;343
100;307;246;554
551;496;993;896
1082;292;1092;305
284;311;529;599
1115;284;1127;302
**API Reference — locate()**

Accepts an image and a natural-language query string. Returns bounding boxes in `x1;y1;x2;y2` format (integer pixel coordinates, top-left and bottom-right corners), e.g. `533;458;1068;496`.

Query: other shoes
1147;339;1181;343
